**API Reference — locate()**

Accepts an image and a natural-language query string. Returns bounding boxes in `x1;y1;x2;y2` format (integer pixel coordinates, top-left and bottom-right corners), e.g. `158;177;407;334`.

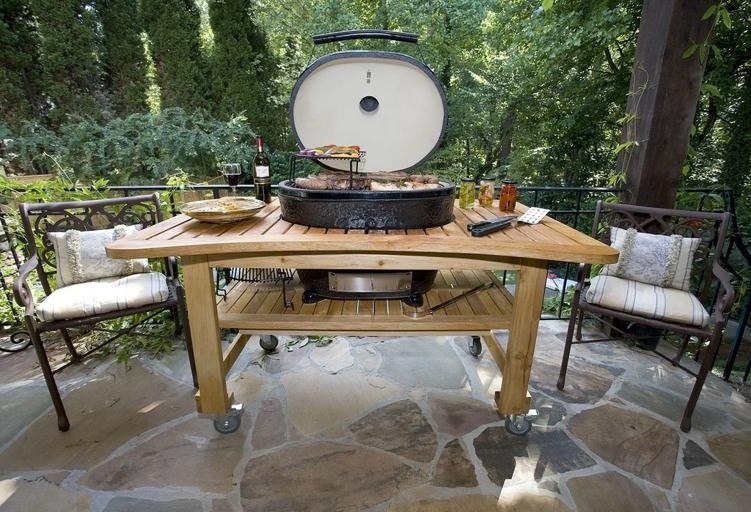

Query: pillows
46;222;151;288
597;225;703;293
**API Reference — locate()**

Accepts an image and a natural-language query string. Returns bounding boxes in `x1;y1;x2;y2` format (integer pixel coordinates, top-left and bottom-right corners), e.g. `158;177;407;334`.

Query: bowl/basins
178;196;268;226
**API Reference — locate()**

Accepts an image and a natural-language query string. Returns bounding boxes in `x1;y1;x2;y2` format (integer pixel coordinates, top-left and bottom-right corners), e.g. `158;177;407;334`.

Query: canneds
459;178;476;208
499;180;518;212
478;177;496;208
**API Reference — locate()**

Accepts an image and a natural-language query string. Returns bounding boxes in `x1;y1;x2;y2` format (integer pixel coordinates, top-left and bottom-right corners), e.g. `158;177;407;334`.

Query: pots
275;28;457;231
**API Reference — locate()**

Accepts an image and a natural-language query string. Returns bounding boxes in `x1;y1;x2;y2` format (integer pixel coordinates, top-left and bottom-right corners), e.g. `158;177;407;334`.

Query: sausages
295;177;327;190
411;175;440;184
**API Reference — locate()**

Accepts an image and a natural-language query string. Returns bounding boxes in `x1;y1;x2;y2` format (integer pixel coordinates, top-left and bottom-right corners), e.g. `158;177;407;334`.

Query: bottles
250;134;273;204
458;175;517;213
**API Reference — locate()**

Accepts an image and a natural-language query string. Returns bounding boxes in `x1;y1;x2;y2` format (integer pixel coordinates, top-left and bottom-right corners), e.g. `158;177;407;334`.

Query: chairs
13;191;199;433
556;199;735;434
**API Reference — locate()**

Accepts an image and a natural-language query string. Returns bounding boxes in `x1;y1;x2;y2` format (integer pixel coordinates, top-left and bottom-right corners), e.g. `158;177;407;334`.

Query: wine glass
222;161;242;199
